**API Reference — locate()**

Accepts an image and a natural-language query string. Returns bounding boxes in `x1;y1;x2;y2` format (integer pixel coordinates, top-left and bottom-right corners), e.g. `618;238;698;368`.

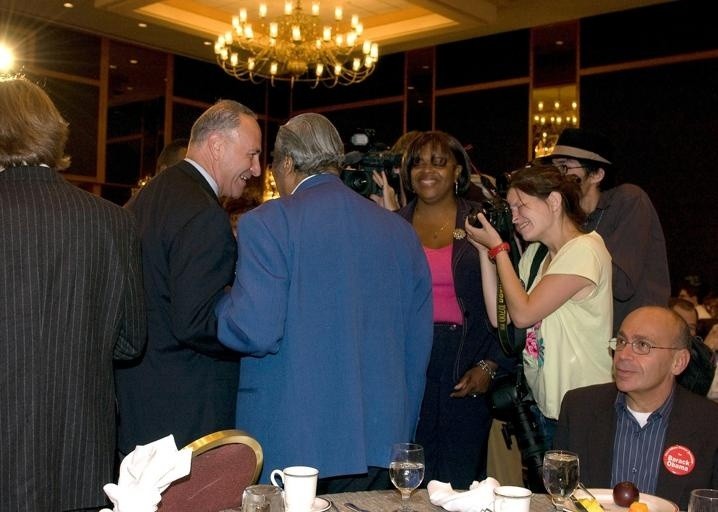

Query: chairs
156;430;264;512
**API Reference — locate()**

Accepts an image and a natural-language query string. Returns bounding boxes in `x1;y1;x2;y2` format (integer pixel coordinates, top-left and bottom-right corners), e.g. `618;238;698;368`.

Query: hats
537;128;614;167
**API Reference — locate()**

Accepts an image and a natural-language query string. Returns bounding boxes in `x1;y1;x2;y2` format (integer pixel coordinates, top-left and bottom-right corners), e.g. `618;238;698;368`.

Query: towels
98;433;194;512
428;476;501;512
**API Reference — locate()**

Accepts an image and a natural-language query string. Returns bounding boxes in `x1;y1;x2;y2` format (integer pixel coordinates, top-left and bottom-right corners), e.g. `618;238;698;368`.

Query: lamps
214;1;380;89
531;88;579;127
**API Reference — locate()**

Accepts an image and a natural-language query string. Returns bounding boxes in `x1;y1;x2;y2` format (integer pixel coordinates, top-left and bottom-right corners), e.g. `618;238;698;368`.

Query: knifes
569;495;589;512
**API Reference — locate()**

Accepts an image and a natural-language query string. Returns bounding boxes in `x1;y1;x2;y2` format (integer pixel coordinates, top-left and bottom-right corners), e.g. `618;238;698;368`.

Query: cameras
489;374;551;480
340;168;378;197
470;199;514;237
496;162;533;198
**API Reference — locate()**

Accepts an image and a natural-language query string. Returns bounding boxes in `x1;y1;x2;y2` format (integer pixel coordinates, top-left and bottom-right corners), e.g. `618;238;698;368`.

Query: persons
117;100;717;512
0;78;148;512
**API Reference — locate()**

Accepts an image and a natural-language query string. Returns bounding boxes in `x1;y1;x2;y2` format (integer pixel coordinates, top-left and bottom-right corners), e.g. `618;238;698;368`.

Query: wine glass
389;443;426;511
542;449;581;512
241;484;284;512
688;488;718;512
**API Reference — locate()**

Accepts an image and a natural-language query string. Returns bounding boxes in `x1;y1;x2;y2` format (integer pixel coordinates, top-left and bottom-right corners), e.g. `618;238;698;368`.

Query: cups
271;465;319;512
493;485;532;512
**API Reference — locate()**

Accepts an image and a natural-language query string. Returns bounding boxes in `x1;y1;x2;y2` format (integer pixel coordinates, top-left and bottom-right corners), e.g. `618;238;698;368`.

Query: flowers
526;318;544;370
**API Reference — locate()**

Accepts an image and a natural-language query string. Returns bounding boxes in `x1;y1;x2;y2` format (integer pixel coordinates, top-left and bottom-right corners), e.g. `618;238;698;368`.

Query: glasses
557;164;584;174
609;337;680;355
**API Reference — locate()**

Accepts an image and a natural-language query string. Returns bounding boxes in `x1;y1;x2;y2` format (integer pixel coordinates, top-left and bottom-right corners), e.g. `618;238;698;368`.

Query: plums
612;481;639;507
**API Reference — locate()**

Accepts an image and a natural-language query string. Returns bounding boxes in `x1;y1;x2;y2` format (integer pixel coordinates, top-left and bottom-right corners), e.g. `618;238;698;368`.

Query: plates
552;488;679;512
283;496;332;512
